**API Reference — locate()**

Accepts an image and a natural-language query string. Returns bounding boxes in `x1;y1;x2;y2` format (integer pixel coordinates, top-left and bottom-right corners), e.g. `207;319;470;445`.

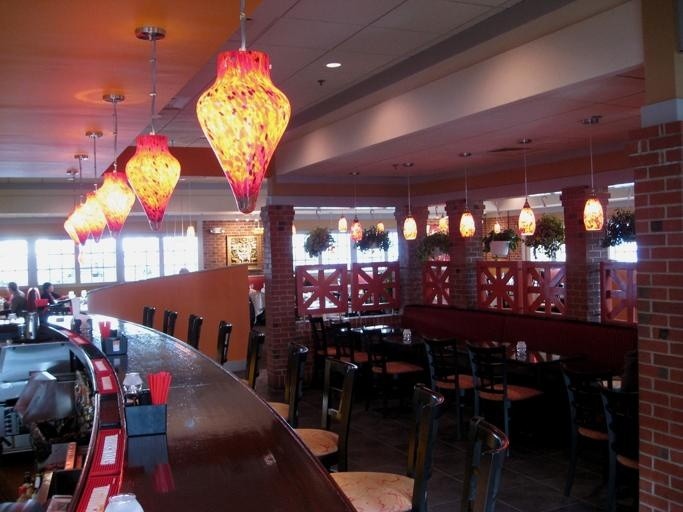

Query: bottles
100;491;146;512
121;372;143;392
3;471;43;511
515;340;526;353
7;313;17;323
402;327;412;341
24;312;39;339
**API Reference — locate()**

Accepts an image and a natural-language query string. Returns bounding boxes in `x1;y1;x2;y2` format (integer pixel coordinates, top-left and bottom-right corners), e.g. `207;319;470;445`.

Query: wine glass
16;324;25;337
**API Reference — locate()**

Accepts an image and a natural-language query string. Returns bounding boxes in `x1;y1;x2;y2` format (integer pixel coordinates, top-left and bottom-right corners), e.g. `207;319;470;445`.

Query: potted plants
600;206;634;248
356;227;388;251
304;227;335;255
526;212;565;256
484;229;520;258
414;233;451;263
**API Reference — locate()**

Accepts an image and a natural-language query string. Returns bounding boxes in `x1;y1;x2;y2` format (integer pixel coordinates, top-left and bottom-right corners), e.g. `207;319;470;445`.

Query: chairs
1;294;82;317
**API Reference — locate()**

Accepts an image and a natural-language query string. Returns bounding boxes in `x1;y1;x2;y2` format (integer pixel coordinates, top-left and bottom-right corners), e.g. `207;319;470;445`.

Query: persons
4;282;26;312
38;282;68;313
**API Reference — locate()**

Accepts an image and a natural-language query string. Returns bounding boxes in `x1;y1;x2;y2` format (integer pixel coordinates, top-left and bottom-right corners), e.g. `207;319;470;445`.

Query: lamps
517;138;537;236
377;219;384;233
350;170;365;241
493;221;500;232
582;118;605;232
404;162;417;241
336;182;347;233
459;148;476;240
